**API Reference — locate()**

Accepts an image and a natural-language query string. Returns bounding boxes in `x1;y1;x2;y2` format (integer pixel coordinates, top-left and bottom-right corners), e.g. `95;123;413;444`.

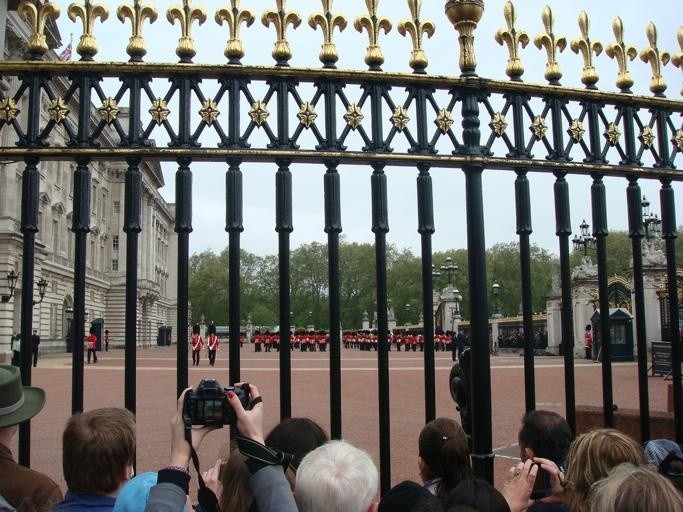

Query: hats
209;325;216;336
0;364;45;428
644;439;682;471
193;324;200;336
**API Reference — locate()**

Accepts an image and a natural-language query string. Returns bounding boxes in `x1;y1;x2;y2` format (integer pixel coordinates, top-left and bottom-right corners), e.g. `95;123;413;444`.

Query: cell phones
530;462;546;499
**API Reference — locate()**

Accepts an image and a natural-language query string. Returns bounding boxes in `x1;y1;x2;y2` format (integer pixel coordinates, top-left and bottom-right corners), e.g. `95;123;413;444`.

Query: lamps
431;256;463;315
289;311;294;318
1;269;20;303
405;303;411;323
32;277;48;306
640;194;663;237
572;219;598;257
308;311;313;325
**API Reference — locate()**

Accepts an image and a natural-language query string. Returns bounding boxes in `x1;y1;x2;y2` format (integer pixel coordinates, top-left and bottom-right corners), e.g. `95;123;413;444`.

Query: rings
513;470;521;480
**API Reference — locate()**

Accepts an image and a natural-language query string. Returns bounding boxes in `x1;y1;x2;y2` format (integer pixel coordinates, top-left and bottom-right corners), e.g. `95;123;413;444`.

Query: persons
49;404;138;511
290;331;329;352
85;331;97;365
374;477;443;512
292;436;381;511
141;375;302;512
503;426;653;511
534;330;546;349
584;327;594;360
584;461;683;512
240;333;244;346
218;445;256;512
110;468;190;511
342;330;378;351
104;332;110;351
204;332;218;367
440;476;511;512
251;330;280;352
191;417;332;512
434;330;470;361
190;329;203;367
643;437;683;494
513;406;573;512
416;415;477;495
388;330;424;351
0;364;65;512
498;332;524;348
29;330;39;367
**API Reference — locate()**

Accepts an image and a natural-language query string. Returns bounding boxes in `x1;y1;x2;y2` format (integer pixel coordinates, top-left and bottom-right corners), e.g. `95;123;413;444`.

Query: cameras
184;378;247;425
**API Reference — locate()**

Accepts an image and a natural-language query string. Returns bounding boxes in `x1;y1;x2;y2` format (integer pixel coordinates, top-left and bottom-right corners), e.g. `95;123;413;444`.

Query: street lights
308;311;312;325
639;193;661;242
404;303;411;323
440;256;458;285
430;264;439;288
289;311;294;327
573;218;597;261
453;290;461;315
386;298;393;311
